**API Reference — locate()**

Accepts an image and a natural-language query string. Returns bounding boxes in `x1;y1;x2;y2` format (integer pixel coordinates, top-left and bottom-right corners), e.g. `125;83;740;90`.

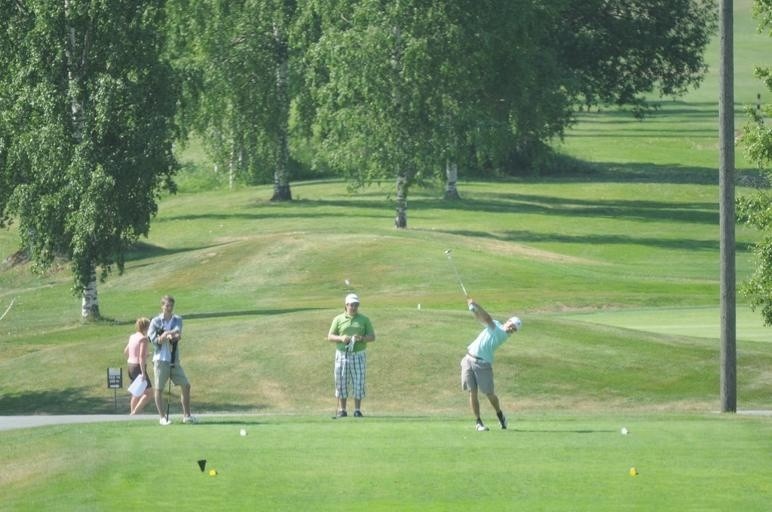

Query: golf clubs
331;342;349;420
165;338;178;426
446;249;473;314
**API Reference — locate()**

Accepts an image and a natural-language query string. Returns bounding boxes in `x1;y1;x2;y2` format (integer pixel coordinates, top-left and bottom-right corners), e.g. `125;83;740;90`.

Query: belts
467;352;484;360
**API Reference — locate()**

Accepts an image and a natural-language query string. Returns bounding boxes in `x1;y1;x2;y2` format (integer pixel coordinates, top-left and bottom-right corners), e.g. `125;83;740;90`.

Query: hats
510;317;521;329
346;294;360;304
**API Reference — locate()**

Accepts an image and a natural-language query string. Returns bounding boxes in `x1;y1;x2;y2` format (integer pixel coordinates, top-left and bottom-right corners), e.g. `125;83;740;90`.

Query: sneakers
337;410;362;417
476;423;489;432
159;416;198;425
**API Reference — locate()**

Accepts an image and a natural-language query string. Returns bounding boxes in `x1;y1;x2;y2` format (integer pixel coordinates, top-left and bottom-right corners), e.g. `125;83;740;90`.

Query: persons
328;293;375;417
461;298;521;431
123;316;153;415
147;295;198;425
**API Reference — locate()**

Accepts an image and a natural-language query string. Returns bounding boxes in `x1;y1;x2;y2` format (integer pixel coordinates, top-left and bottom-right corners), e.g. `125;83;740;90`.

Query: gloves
345;335;356;352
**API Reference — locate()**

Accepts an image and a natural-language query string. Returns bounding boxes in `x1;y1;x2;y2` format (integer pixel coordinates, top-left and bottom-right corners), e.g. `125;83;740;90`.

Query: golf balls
239;428;247;437
620;428;630;436
208;468;217;479
629;467;638;477
